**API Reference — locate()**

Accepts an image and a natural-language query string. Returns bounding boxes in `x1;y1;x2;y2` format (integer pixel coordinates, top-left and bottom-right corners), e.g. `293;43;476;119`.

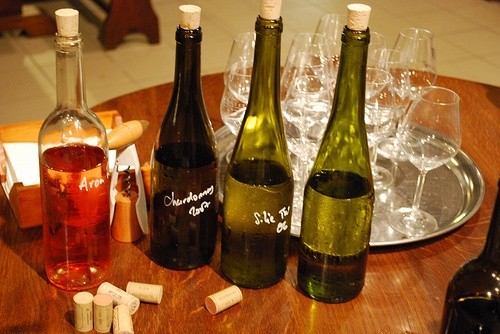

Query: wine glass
280;14;340;227
365;26;461;237
219;28;256;164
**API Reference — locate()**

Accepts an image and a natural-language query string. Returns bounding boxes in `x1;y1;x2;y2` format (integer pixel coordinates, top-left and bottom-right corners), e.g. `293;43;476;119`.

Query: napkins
2;142;148;235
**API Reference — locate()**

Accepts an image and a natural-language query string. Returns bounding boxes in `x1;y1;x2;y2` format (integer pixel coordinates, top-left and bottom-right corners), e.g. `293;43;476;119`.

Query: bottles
112;165;142;245
439;177;500;334
149;5;220;271
39;8;112;292
296;3;375;304
219;0;294;289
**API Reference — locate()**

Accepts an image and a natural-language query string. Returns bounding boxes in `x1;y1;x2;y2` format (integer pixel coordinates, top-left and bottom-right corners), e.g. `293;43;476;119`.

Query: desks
0;68;500;334
